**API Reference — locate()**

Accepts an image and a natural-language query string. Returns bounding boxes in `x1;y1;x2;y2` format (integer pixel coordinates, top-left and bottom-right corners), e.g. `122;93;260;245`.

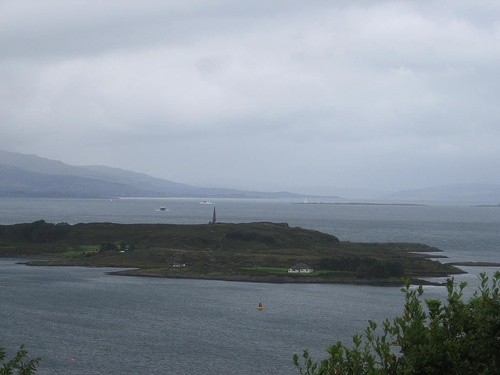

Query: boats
156;206;169;211
199;200;215;205
